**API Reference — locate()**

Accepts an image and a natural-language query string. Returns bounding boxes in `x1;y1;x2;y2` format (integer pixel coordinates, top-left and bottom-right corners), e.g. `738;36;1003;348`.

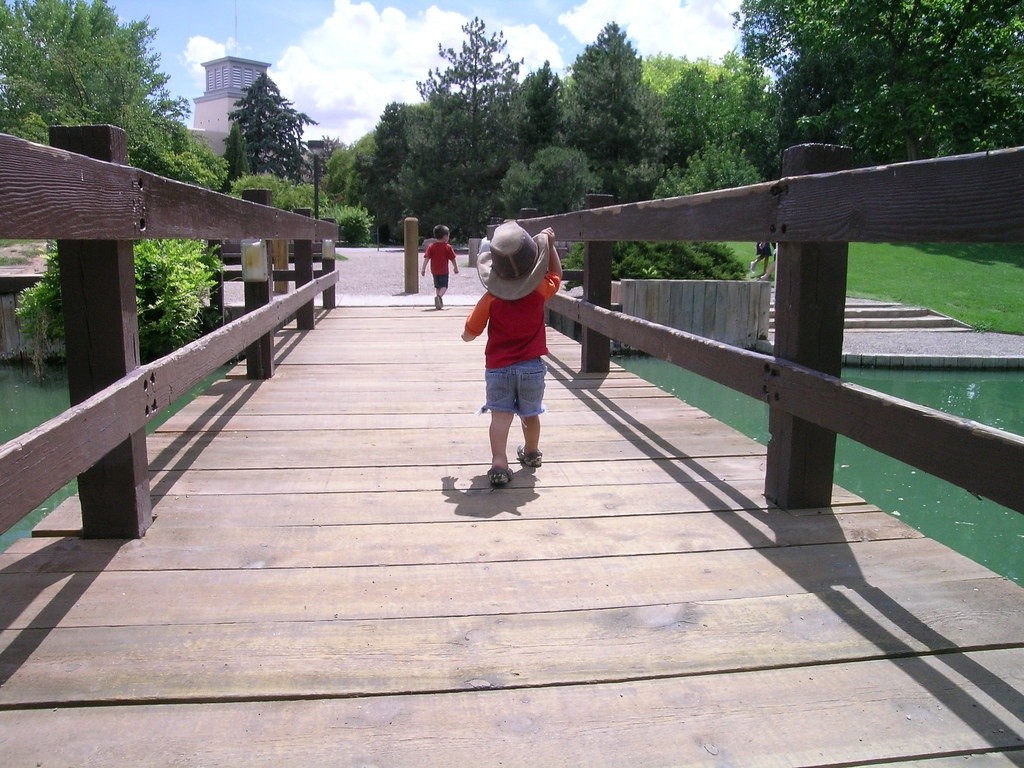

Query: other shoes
434;295;444;310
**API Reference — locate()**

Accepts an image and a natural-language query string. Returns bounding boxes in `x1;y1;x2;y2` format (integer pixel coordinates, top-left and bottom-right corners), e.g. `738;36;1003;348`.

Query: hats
477;221;549;301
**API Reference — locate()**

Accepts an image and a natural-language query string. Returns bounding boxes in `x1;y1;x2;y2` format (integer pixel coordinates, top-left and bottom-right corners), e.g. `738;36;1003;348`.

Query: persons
462;218;562;486
751;241;777;280
421;225;459;310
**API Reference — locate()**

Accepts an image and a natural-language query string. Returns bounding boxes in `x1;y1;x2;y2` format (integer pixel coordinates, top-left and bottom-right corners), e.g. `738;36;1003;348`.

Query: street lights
308;139;324;220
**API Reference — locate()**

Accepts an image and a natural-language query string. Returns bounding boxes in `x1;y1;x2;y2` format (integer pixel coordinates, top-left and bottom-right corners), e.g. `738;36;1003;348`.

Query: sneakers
517;444;542;467
487;466;514;485
